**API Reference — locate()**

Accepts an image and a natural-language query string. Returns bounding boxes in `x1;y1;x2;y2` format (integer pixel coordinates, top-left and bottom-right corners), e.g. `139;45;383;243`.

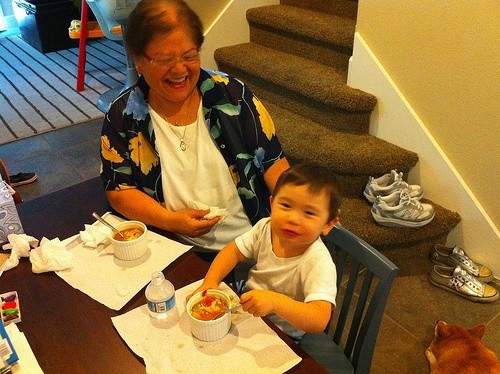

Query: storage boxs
12;0;81;54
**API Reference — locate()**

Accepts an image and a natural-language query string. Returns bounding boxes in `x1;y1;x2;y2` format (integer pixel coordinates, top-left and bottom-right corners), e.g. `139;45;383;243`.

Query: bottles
144;270;180;330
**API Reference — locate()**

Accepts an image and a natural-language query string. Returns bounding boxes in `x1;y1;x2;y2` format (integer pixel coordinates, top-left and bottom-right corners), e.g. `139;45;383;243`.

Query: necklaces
153;88;192;151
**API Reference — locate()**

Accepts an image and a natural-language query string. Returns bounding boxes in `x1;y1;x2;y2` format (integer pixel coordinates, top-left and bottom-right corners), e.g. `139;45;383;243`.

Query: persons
99;0;292;292
186;160;344;345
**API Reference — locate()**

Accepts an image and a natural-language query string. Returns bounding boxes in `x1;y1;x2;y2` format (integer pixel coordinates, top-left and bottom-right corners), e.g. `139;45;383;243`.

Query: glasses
142;52;200;70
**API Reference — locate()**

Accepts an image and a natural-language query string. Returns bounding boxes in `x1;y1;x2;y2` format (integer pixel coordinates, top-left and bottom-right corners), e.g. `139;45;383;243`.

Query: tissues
0;183;24;242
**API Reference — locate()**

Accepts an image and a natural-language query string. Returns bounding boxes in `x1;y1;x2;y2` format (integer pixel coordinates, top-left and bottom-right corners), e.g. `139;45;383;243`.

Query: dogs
425;321;500;374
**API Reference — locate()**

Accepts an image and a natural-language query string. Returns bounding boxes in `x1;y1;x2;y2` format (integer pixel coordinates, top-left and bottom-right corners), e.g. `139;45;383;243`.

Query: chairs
299;226;399;374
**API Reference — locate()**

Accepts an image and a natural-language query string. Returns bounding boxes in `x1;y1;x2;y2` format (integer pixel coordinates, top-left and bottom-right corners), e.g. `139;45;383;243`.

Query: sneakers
370;189;435;228
362;169;424;207
427;265;500;303
428;243;494;283
9;172;38;186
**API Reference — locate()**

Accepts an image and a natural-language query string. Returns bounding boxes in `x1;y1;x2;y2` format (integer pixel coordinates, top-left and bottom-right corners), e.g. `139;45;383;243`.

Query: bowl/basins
110;219;148;260
187;289;233;342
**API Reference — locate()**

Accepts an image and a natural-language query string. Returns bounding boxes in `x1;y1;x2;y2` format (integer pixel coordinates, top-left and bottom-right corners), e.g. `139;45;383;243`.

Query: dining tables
0;175;332;374
